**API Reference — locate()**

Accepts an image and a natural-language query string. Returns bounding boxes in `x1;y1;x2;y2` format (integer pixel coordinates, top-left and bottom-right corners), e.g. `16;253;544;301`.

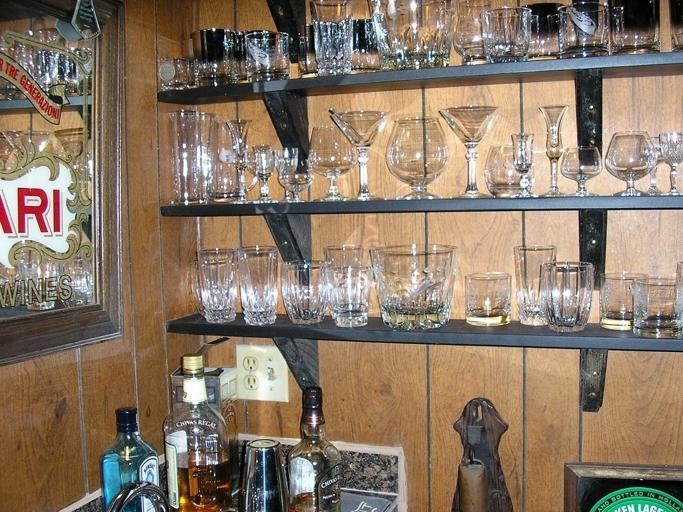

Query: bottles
158;350;234;512
285;383;342;512
99;406;162;511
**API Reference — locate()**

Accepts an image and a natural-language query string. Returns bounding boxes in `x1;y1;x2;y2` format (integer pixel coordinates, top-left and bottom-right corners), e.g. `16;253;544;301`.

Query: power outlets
234;346;290;408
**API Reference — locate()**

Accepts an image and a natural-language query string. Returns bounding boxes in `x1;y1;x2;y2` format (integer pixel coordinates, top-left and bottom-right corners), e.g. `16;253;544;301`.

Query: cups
323;246;362;322
542;262;594;333
196;248;237;323
209;120;244;202
512;244;558;327
674;261;683;340
1;25;91;101
239;436;286;512
1;243;92;310
367;243;458;331
237;246;279;325
630;277;676;337
599;272;649;333
280;260;326;324
463;269;512;329
161;0;682;88
162;108;213;203
328;263;373;329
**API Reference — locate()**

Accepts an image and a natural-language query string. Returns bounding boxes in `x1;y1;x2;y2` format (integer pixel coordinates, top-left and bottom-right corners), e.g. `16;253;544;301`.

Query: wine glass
561;147;604;196
645;134;664;195
658;131;682;194
277;145;313;204
386;115;449;198
328;105;390;202
0;127;88;205
604;131;656;197
438;105;499;199
536;104;572;197
507;131;543;197
484;145;533;197
308;124;362;201
247;143;280;206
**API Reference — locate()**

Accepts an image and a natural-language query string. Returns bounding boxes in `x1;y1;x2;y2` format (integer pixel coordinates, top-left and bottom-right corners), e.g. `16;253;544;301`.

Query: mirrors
1;1;128;369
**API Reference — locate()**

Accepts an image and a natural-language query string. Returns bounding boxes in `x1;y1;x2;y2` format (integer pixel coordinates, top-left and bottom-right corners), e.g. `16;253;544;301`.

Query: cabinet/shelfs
155;51;683;415
1;93;94;321
